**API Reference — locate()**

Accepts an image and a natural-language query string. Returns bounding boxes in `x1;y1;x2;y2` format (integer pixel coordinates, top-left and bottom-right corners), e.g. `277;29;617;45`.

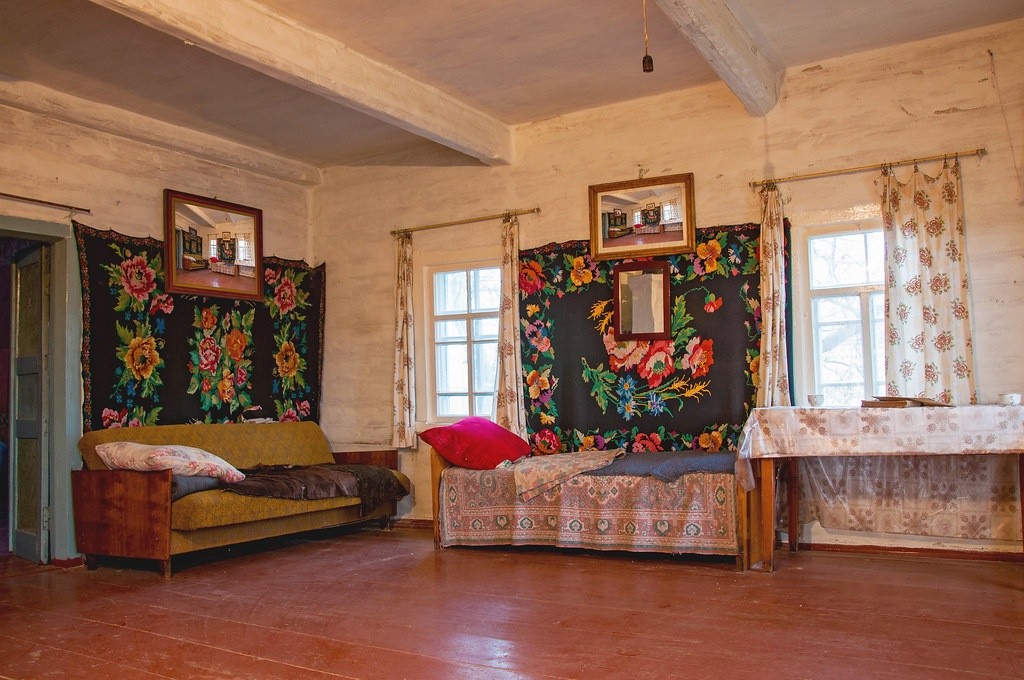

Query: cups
997;393;1021;405
808;395;824;407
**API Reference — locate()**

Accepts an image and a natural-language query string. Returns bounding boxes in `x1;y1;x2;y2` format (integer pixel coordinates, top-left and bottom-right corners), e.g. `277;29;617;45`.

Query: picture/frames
189;227;197;242
588;172;696;262
163;187;265;305
646;203;655;211
614;209;622;218
222;232;231;241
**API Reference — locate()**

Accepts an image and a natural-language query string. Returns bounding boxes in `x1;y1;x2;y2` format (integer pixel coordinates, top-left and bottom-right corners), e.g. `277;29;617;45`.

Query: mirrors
614;261;672;342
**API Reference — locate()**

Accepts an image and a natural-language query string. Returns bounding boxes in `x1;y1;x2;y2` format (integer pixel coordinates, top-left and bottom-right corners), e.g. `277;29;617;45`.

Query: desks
235;259;256;279
660;219;683;232
734;402;1024;577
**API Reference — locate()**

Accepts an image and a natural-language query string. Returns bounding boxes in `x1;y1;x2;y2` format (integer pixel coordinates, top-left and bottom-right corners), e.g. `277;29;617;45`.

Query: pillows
184;255;197;263
93;440;247;485
210;257;221;263
634;224;643;228
416;416;535;470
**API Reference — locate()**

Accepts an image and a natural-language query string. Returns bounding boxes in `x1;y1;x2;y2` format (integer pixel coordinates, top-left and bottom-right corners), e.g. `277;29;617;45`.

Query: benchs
182;253;209;271
635;225;664;235
609;225;628;240
429;446;762;574
208;262;239;276
70;420;414;579
